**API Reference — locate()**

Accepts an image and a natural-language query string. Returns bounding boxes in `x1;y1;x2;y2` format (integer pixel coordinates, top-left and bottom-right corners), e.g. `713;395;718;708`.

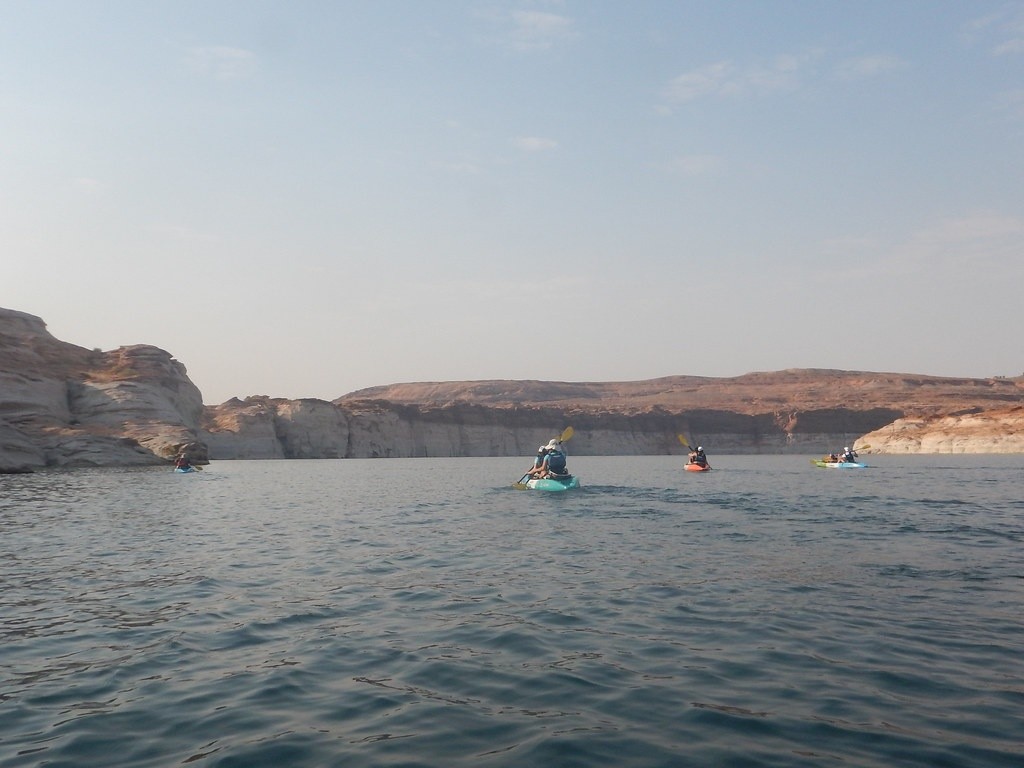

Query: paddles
189;462;204;470
512;425;573;487
678;434;714;470
520;436;561;490
811;444;871;464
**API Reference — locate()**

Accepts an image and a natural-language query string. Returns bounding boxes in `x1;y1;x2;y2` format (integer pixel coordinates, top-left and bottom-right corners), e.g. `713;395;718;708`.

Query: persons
688;446;706;468
826;447;858;463
526;439;566;479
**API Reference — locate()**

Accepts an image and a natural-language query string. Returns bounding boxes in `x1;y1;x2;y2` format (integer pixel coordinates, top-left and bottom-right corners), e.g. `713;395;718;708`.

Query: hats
844;447;849;451
180;453;187;458
698;447;703;452
539;445;545;452
545;439;561;451
